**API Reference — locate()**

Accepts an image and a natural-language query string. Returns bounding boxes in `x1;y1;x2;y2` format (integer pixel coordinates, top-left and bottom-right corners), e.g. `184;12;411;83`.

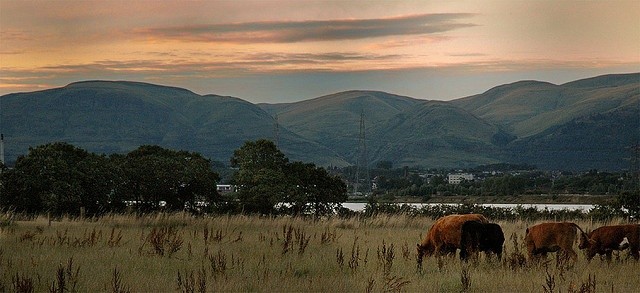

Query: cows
578;224;640;268
416;214;493;264
522;222;596;271
